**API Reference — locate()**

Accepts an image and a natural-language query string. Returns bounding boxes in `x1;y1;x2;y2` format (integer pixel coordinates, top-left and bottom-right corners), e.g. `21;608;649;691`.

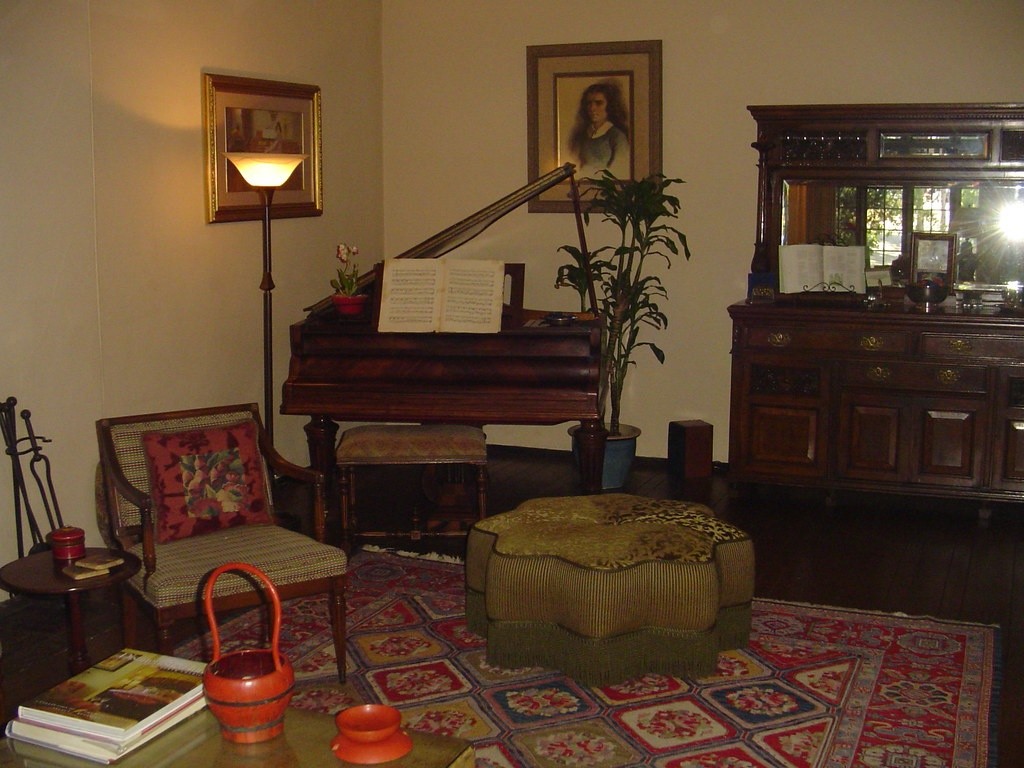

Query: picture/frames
526;39;663;215
909;231;957;297
202;73;323;225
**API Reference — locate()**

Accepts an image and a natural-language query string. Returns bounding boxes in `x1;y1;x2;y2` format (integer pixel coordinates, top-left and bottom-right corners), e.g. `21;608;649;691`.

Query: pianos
280;162;611;542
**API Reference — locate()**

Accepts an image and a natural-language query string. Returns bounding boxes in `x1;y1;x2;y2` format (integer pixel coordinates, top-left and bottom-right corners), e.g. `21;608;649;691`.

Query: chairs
96;402;348;685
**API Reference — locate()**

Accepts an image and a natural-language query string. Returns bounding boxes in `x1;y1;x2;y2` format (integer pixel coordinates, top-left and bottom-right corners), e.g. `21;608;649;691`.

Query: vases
333;295;366;314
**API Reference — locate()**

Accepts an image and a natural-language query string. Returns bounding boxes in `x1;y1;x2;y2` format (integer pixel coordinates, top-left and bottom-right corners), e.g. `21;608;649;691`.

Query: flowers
329;243;360;295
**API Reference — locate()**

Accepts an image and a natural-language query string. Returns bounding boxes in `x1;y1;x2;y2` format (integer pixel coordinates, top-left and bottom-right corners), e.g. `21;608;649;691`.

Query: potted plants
555;170;691;490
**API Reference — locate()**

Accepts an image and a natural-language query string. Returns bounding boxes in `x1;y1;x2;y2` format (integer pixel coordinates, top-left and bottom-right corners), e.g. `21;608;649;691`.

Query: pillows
142;420;274;544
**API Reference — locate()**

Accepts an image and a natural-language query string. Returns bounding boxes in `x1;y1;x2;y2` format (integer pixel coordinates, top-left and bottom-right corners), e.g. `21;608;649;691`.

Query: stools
465;492;755;689
336;424;487;561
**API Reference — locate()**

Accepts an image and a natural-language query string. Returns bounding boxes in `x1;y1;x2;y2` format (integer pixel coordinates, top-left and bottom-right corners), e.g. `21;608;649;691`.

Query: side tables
0;549;143;663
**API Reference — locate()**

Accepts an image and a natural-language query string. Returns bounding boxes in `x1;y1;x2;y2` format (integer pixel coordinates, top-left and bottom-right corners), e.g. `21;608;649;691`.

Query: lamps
947;207;984;282
219;152;311;450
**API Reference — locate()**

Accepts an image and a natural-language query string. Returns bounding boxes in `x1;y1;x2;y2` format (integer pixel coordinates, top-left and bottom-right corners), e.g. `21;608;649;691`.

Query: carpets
157;542;1006;768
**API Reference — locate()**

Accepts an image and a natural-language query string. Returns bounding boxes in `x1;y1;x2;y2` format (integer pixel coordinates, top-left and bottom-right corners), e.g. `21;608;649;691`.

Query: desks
0;704;475;768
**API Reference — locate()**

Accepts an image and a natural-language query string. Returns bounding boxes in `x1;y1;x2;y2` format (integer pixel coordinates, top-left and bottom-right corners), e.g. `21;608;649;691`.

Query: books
4;649;210;765
779;242;866;295
61;565;109;580
378;257;504;334
75;554;125;569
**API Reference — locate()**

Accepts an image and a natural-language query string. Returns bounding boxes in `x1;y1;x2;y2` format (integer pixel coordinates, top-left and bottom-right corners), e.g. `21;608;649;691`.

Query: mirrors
773;175;1023;311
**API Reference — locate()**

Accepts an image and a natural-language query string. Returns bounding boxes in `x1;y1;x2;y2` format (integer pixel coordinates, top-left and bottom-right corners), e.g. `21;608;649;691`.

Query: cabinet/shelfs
727;298;1024;504
